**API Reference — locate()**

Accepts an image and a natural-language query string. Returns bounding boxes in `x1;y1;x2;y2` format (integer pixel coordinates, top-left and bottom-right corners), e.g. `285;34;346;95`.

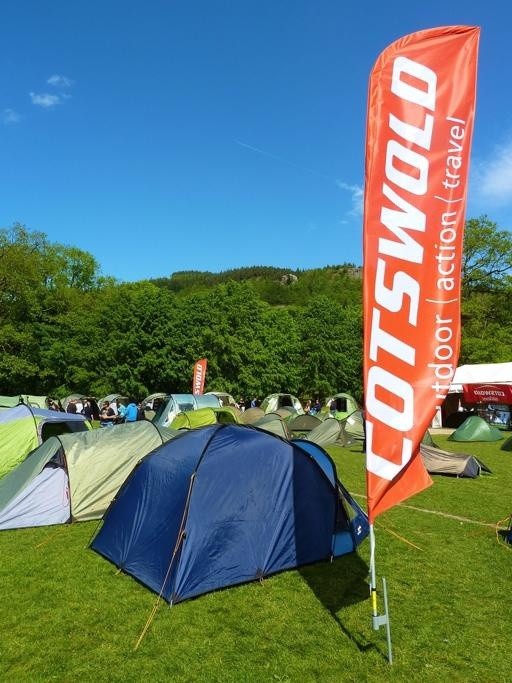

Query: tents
0;391;512;608
